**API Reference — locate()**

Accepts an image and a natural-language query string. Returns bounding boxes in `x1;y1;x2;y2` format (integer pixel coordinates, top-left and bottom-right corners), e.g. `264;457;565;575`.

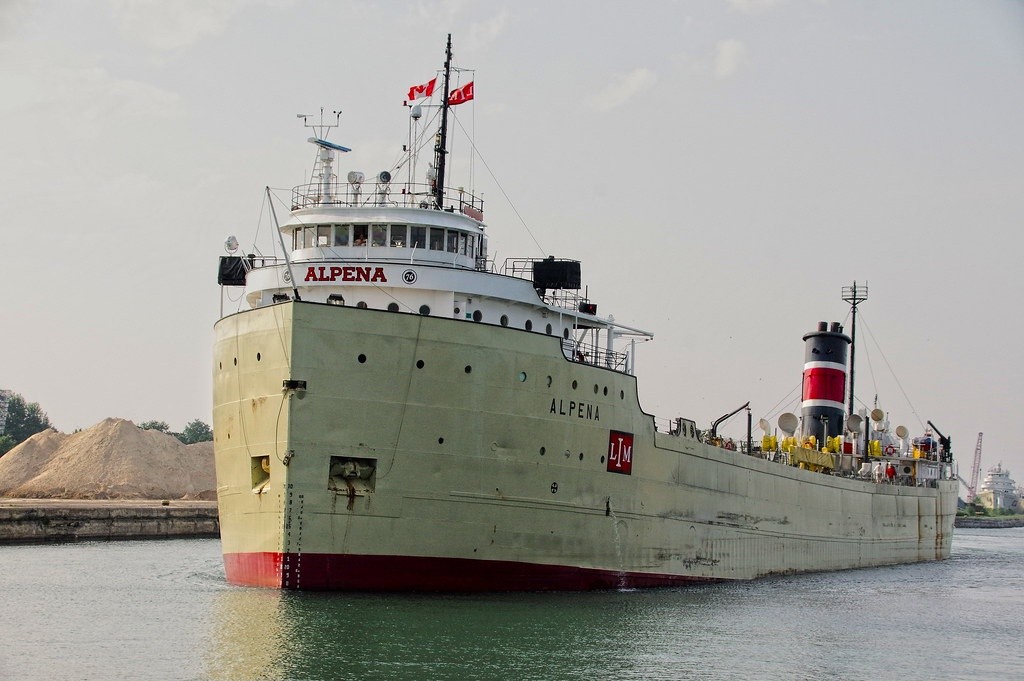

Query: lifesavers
885;445;895;456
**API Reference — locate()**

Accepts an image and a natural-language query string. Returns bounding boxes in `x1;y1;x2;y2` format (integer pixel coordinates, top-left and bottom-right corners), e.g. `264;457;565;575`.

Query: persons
354;233;367;246
873;463;884;484
887;464;895;485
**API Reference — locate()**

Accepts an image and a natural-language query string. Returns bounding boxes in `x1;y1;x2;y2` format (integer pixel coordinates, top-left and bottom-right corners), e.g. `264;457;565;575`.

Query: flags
405;73;442;101
447;81;474;105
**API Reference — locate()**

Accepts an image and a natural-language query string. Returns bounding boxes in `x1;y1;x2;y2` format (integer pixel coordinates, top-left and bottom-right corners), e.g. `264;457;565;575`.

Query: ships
211;32;959;596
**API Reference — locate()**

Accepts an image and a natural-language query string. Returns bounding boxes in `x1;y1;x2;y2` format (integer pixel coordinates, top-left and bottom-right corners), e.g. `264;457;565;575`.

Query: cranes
968;431;984;503
